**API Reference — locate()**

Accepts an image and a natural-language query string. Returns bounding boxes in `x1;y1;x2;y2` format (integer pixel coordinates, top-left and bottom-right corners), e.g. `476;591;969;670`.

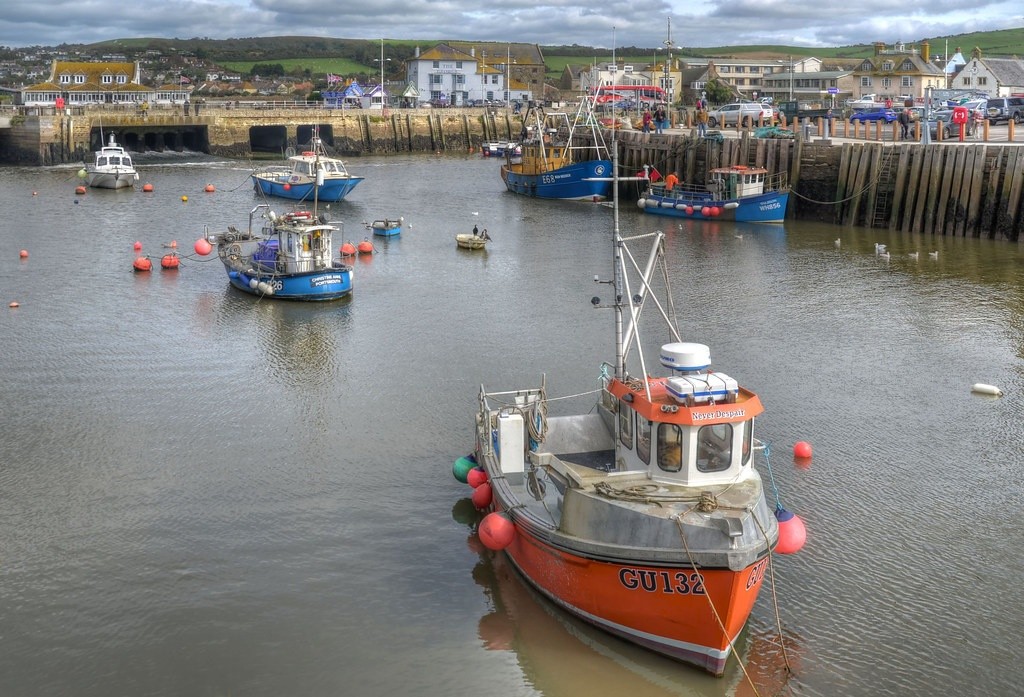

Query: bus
590;85;667;110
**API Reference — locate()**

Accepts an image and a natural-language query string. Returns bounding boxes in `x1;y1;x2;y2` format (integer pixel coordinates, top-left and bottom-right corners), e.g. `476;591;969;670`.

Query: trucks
777;100;831;126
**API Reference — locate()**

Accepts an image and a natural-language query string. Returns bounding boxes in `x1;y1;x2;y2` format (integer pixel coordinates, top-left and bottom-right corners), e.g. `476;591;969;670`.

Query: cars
960;101;988;121
848;107;897;126
474;99;505;107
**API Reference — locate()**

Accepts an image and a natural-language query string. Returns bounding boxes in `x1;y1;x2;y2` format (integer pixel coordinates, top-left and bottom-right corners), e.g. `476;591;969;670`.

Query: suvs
985;96;1024;125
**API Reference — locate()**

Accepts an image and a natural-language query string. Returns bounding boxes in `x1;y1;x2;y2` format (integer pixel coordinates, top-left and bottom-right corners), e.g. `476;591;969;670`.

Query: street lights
707;103;773;128
480;65;487;103
373;58;392;109
656;46;683;119
935;58;957;89
776;56;793;102
501;61;517;107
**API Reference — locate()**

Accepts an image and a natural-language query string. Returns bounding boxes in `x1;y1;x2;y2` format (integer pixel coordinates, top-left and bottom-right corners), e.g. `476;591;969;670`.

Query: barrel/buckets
679;124;683;129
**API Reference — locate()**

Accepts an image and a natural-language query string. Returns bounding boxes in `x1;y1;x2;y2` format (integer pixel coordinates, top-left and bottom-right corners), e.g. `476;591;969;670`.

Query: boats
372;219;402;237
84;130;136;190
453;139;807;679
909;109;974;140
482;141;522;157
637;164;792;225
499;88;612;203
217;138;354;302
250;113;366;204
456;228;489;250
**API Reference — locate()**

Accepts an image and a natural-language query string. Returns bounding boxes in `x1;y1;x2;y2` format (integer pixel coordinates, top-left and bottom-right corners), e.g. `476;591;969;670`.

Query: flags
327;73;342;83
649;169;661;181
181;76;190;83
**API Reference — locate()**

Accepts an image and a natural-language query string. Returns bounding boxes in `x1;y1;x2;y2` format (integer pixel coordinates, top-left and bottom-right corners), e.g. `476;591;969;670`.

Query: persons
184;99;190;116
141;101;148;116
697;99;706;109
899;109;909;140
665;171;679;196
824;110;834;134
971;110;982;139
653;106;666;134
194;99;201;116
697;108;709;137
642;108;652;133
